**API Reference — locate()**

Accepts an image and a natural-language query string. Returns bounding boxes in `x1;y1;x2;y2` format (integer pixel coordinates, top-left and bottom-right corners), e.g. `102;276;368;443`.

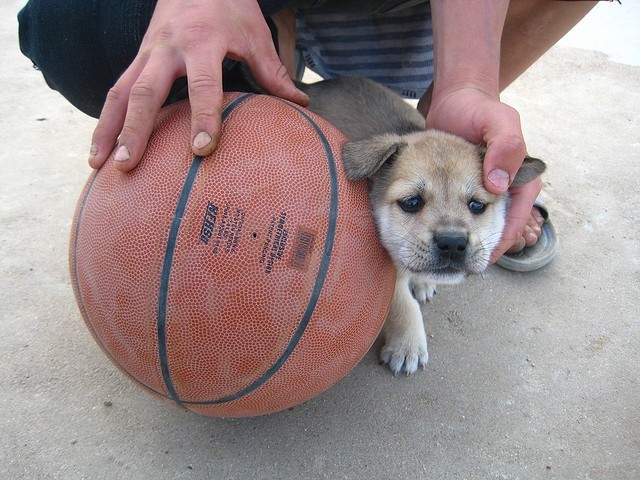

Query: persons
16;0;606;275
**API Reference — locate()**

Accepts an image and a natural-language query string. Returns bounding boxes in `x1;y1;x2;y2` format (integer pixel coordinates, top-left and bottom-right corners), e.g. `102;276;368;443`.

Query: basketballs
68;90;398;418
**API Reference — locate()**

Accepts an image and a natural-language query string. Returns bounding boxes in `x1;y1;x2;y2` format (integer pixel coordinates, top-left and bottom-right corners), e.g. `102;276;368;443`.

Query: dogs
238;71;547;378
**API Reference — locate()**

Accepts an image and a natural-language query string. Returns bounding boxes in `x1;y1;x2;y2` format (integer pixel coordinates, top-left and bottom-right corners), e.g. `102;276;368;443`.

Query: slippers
496;196;558;272
